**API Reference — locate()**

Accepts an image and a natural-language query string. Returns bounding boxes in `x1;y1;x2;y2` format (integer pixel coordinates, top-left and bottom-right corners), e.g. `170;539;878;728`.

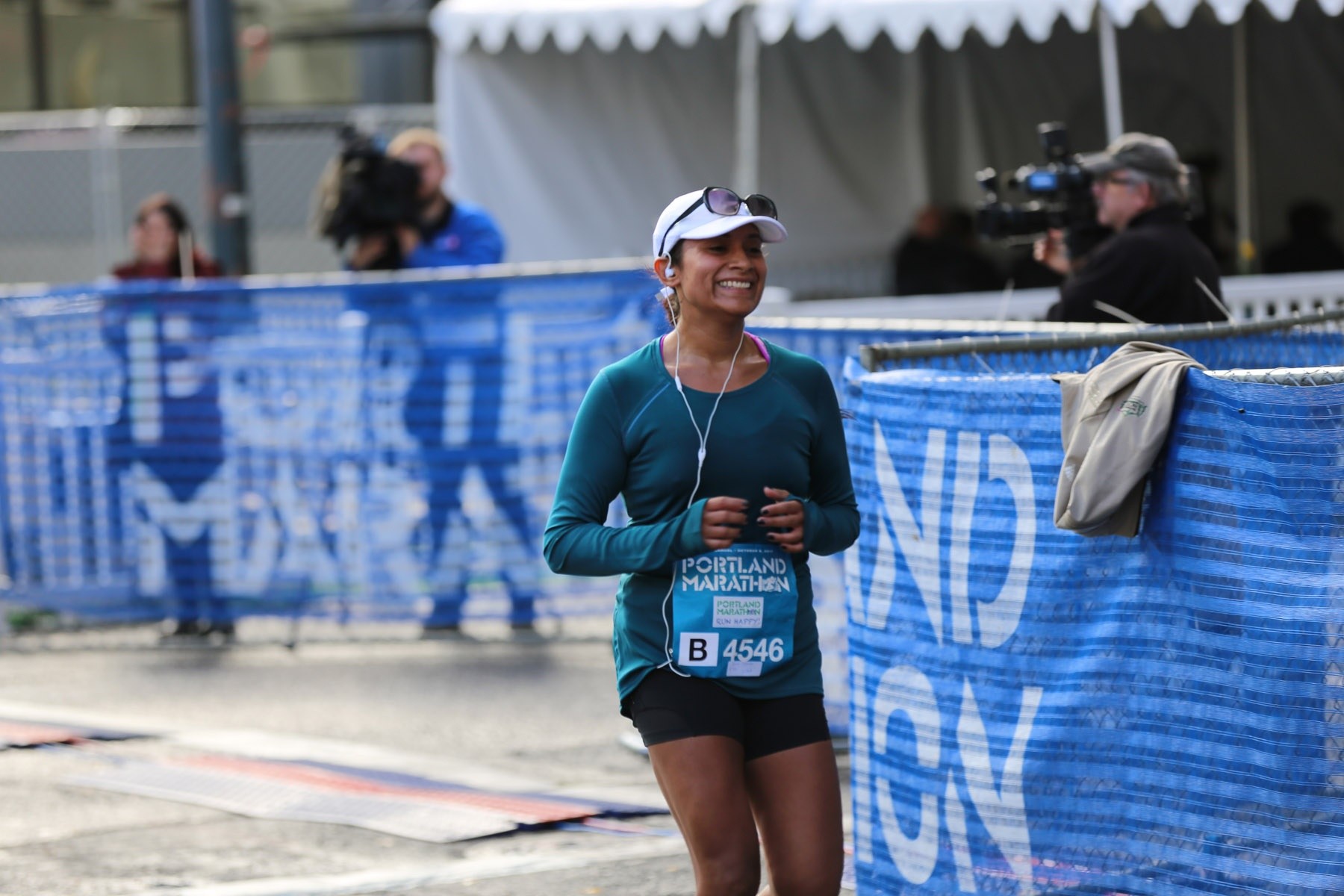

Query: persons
892;129;1230;326
541;185;862;896
97;193;242;648
340;132;553;642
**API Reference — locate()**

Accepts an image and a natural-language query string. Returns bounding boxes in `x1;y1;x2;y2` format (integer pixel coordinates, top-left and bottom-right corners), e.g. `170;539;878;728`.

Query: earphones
665;262;675;278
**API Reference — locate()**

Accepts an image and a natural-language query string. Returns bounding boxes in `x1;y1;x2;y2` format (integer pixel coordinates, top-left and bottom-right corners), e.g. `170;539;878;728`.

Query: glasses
1095;171;1137;187
658;186;778;257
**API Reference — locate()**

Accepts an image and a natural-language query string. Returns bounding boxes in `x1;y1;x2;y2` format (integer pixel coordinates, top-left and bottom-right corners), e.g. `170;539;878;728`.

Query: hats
653;188;788;260
1073;131;1190;185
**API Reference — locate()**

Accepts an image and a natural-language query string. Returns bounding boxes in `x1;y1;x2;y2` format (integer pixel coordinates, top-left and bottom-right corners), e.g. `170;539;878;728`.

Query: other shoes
173;624;200;636
208;608;234;635
